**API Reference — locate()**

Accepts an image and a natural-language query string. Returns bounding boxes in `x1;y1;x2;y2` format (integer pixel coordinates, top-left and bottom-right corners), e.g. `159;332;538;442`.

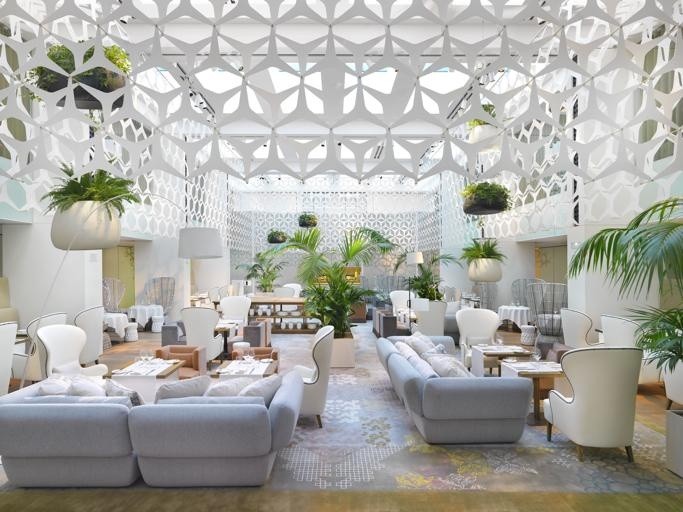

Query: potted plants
31;40;134;110
461;184;509;214
462;240;498;280
299;215;317;227
267;230;286;243
468;105;499;147
570;198;683;479
42;162;142;251
272;227;401;367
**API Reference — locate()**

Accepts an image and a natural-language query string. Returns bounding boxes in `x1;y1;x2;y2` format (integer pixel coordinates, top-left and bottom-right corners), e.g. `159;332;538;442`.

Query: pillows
405;331;476;379
36;374;280;406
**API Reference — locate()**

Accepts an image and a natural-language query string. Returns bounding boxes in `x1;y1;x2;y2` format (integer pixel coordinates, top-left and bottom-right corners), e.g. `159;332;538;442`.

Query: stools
521;325;535;346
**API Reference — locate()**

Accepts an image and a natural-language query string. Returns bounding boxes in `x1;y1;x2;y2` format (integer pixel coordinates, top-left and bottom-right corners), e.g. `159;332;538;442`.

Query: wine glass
140;350;154;368
241;347;256;366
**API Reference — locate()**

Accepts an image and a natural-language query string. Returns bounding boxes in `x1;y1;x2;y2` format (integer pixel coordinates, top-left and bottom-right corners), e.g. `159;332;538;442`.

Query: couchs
374;291;479;336
162;296;278;373
378;335;534;446
287;325;336;428
0;371;304;490
542;308;683;461
456;308;503;376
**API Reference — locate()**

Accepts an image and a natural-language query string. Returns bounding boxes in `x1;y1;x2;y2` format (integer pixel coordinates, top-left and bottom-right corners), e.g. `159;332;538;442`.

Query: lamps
407;252;436;300
19;192;220;389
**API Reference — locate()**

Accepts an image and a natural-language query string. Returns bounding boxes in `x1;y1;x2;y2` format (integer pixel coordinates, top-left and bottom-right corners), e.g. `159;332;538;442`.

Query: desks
104;305;163;343
499;306;531;331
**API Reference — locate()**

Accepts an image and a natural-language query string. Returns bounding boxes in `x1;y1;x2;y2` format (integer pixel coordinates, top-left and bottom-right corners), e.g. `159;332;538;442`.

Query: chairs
1;304;103;395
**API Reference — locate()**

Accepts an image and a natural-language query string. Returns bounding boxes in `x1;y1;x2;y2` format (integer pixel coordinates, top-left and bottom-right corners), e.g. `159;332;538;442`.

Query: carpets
83;318;683;494
